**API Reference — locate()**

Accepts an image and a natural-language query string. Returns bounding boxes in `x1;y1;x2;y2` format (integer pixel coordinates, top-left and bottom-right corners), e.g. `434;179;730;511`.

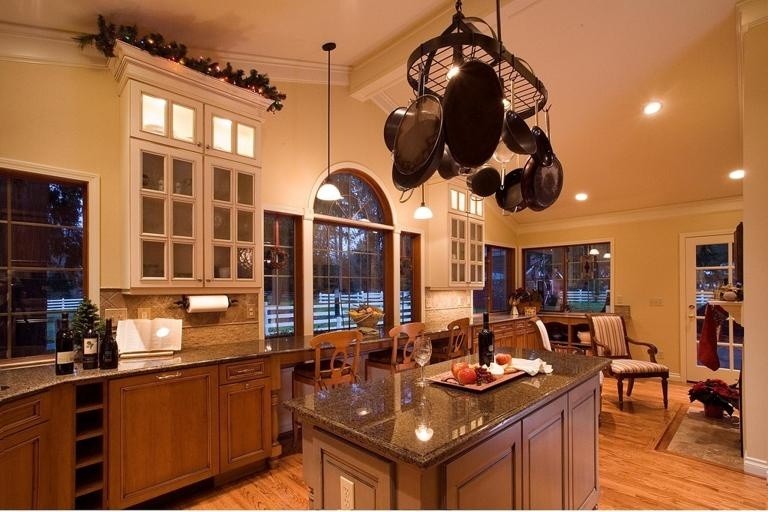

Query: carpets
648;403;744;472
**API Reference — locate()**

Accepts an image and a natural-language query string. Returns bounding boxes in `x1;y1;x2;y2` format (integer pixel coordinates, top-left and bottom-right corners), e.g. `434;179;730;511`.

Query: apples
495;352;512;365
458;367;477;385
451;361;470;379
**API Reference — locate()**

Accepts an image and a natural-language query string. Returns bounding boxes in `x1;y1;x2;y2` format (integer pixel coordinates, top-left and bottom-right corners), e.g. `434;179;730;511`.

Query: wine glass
414;384;431;428
414;336;432;384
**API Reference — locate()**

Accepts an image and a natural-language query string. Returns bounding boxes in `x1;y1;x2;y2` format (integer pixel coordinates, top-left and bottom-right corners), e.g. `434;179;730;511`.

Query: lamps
415;181;433;219
416;395;434;442
316;43;341;201
589;244;600;255
603;244;610;259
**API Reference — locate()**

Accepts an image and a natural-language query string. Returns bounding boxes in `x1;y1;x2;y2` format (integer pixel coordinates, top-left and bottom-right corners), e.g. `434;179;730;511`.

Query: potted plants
72;295;107;364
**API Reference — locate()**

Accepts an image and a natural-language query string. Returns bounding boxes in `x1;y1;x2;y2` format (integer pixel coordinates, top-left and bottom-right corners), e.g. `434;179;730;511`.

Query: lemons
358;306;380;317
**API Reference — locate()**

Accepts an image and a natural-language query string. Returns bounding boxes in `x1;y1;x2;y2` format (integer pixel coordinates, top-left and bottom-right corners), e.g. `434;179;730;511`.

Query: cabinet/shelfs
0;376;108;510
107;38;275;296
109;358;220;510
424;169;485;291
537;312;594;356
708;299;744;327
219;351;272;489
515;316;542;353
472;319;514;351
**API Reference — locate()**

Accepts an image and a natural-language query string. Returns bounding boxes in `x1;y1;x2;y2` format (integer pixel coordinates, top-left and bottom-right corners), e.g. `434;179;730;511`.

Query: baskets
350;308;385;327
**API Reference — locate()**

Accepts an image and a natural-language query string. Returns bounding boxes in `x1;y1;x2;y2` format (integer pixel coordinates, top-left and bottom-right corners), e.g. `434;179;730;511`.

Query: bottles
100;318;118;369
82;315;98;369
478;312;494;367
56;313;74;375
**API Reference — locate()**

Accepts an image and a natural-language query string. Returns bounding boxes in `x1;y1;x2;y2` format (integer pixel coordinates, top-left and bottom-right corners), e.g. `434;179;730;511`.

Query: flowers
508;287;543;312
686;379;740;415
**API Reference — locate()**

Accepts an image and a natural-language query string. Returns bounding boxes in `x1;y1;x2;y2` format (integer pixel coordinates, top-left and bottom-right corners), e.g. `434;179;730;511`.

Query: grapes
474;366;497;386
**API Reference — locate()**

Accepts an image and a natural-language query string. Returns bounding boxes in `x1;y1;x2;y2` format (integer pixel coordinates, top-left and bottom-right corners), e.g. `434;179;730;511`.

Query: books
115;319;182;359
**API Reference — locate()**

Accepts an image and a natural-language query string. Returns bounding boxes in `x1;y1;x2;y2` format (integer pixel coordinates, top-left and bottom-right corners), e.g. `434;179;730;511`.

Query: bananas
348;309;363;317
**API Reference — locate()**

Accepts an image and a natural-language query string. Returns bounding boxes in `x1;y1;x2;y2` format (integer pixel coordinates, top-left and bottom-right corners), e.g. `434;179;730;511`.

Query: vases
704;403;723;418
523;307;536;316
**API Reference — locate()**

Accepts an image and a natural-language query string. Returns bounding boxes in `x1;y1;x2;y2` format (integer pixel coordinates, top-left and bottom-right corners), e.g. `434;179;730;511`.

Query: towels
511;358;554;377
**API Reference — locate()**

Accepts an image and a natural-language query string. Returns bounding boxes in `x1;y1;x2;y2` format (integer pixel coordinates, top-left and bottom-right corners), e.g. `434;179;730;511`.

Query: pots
495;168;527;216
395;72;443;175
469;167;500;201
505;80;537;154
392;136;445;203
443;61;504;167
438;145;459;180
522;109;563;211
531;101;553;167
384;107;407;153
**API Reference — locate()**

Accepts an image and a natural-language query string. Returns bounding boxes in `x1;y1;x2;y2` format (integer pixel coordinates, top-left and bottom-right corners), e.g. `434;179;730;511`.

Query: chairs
293;330;362;448
528;316;603;427
430;317;469;366
585;314;669;411
365;322;425;380
